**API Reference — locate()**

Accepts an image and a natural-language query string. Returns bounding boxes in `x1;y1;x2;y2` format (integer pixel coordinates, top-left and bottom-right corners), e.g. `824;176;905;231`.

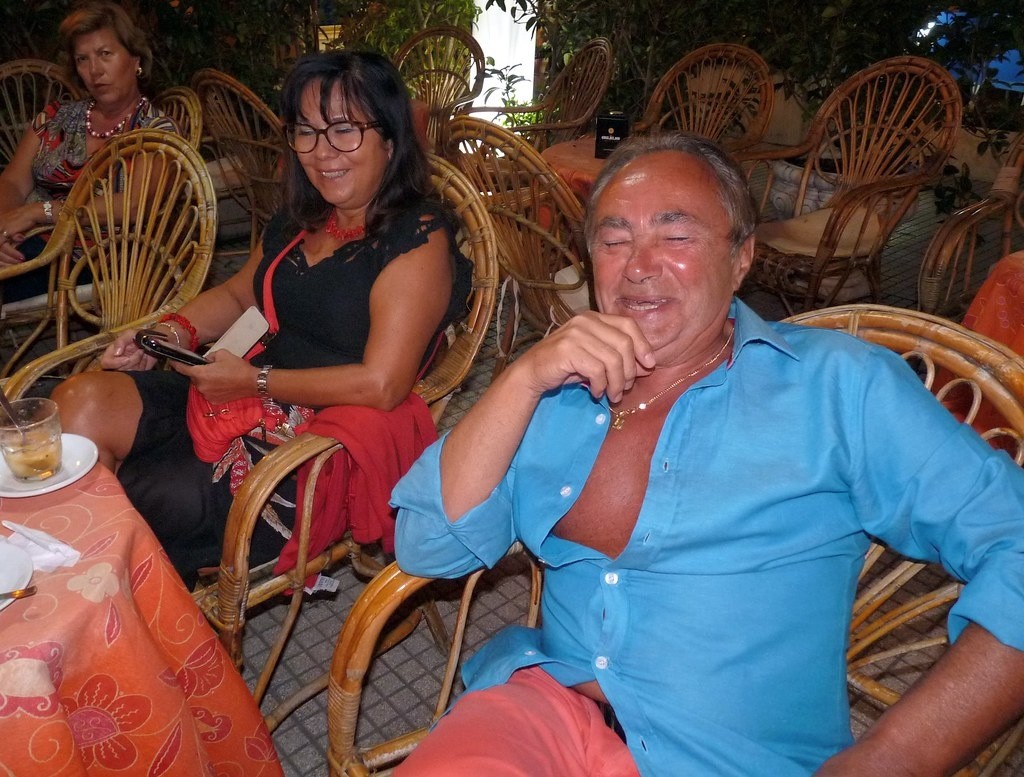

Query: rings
1;230;11;242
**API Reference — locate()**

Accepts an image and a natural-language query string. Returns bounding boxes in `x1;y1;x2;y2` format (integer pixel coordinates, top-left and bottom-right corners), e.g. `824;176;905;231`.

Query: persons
386;129;1024;777
33;45;474;593
0;1;182;303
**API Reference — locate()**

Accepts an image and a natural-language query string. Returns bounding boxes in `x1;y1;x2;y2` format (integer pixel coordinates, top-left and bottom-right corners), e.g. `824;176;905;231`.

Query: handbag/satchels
186;377;289;464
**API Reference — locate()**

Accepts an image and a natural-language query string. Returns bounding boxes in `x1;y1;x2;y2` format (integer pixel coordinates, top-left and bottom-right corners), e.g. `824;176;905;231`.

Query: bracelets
158;323;180;348
160;313;200;353
257;364;273;397
43;201;53;225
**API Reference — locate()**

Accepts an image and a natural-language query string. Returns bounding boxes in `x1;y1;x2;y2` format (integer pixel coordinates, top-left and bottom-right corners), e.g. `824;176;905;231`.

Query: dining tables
0;427;284;777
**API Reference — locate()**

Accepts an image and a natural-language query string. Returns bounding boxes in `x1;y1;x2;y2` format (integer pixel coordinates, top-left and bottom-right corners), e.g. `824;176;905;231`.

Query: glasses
282;120;383;154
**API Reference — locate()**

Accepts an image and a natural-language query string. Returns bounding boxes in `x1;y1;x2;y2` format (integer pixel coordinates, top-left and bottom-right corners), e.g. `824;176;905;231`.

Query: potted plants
725;0;871;148
907;0;1024;185
741;0;943;222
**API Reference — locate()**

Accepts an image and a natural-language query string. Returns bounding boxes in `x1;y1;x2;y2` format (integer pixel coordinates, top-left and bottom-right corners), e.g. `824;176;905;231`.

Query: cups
0;397;64;484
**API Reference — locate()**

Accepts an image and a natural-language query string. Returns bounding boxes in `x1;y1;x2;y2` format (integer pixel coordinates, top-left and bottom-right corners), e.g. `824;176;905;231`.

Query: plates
0;541;33;610
0;433;99;499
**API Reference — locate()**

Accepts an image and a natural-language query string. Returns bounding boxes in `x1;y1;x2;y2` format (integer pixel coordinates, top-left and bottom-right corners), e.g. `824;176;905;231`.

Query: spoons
0;385;49;470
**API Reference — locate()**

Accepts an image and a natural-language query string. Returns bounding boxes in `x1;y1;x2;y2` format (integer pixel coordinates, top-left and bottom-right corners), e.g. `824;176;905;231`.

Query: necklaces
609;328;735;430
325;207;364;240
86;100;133;138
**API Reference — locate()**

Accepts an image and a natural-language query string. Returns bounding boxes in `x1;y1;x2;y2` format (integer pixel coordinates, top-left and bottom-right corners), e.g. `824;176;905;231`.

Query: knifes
0;586;37;598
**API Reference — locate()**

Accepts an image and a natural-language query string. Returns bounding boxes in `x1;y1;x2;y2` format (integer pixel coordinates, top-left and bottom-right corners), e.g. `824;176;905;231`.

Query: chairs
0;0;1024;777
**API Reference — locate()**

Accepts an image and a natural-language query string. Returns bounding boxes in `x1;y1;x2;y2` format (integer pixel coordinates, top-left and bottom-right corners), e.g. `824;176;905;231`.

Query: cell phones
142;336;210;366
